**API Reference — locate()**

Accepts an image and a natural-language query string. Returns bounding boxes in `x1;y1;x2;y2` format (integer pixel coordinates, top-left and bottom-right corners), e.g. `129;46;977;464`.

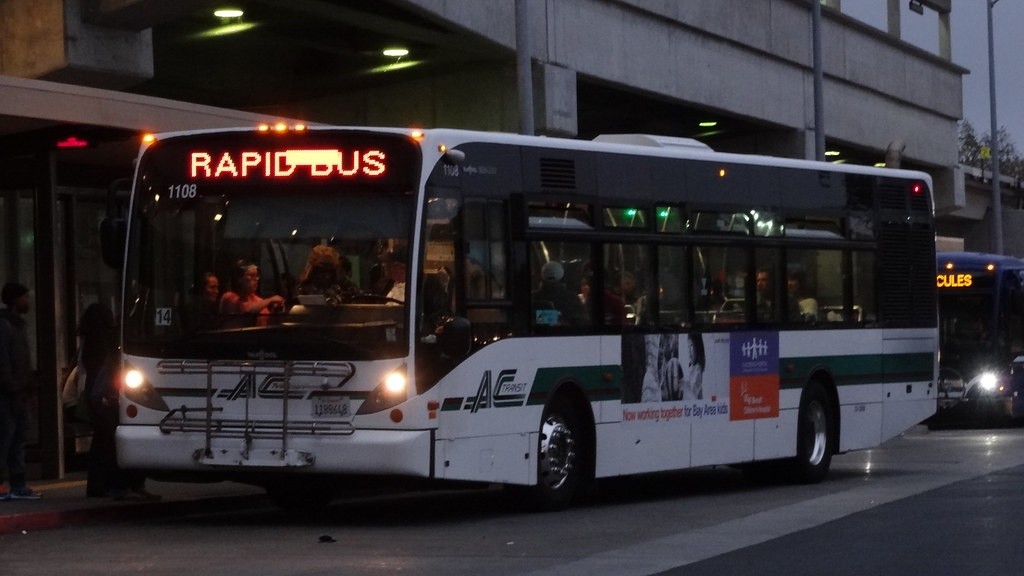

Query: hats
378;244;407;264
540;260;563;282
2;281;28;304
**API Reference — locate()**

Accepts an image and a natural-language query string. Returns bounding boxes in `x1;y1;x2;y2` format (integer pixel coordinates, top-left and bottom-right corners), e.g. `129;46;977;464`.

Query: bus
107;126;942;510
925;251;1023;425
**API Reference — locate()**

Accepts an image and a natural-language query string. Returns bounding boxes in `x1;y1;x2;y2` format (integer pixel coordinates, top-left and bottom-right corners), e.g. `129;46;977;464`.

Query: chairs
422;303;865;345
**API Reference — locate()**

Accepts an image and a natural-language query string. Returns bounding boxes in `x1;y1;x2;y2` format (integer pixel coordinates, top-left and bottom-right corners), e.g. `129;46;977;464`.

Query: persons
195;260;284;315
532;261;636;325
293;245;358;303
0;283;42;500
378;237;430;303
756;267;819;322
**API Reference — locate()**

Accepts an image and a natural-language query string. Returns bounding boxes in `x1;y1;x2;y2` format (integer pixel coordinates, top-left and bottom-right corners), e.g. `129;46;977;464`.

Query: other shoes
109;489;136;500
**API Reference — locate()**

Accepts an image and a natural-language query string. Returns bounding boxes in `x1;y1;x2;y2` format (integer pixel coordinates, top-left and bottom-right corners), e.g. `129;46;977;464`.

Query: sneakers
1;486;41;502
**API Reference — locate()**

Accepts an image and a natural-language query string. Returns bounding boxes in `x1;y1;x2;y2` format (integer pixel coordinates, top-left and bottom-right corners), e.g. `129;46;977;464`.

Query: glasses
239;275;260;281
